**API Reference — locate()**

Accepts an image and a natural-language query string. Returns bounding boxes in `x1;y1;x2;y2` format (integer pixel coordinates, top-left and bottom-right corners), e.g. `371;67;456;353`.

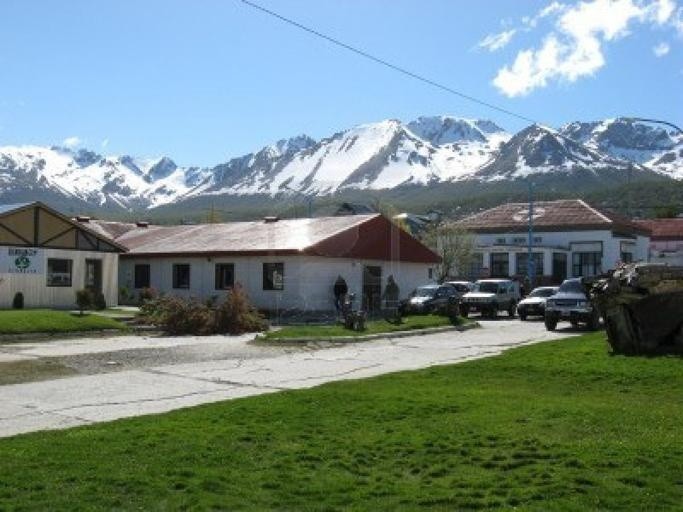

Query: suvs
400;274;610;336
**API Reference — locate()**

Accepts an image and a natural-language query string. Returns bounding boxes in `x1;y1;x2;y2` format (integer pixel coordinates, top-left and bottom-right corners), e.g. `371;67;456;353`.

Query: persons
380;274;399;317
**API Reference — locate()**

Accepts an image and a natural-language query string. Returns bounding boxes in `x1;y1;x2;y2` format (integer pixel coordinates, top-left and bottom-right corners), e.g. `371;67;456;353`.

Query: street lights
281;184;312;219
514;175;535;291
